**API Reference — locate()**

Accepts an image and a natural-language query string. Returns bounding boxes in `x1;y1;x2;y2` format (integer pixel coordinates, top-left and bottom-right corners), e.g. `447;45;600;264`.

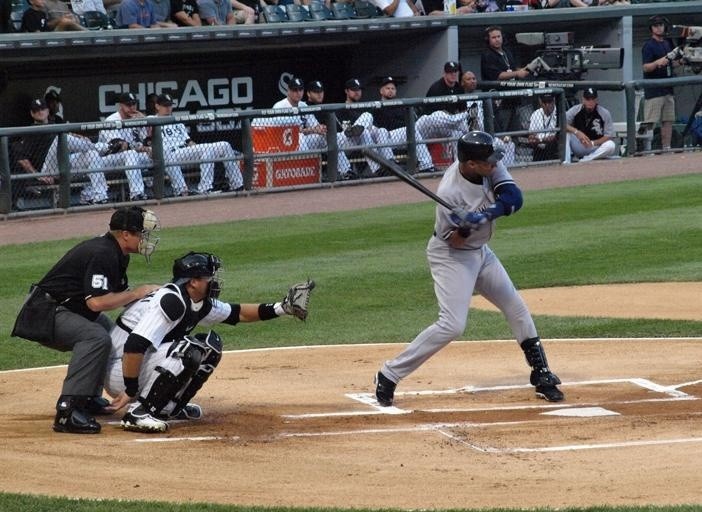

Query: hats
45;90;60;101
30;99;48;111
583;87;598;98
343;78;366;90
444;61;459;73
380;78;398;89
308;81;324;89
119;92;138;104
539;93;555;103
156;93;176;106
288;78;305;89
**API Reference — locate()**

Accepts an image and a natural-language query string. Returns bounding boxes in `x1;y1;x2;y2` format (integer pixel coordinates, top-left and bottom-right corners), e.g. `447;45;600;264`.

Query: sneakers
81;394;113;417
420;166;444;173
535;384;564;402
99;143;123;156
340;171;362;180
95;198;115;205
373;371;397;407
150;403;202;419
344;125;364;137
131;194;152;201
120;412;170;434
52;407;102;434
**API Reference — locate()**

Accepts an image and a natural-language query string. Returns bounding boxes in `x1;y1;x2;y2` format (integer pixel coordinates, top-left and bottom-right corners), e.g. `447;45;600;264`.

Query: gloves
449;199;469;228
464;213;488;231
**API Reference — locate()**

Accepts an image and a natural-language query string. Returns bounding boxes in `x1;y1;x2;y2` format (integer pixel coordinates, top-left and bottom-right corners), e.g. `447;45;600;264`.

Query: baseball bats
362;147;458;212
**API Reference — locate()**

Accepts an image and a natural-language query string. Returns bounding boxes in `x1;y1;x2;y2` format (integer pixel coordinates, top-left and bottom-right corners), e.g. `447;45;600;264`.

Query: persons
101;250;316;434
10;206;159;433
3;1;702;212
373;128;564;407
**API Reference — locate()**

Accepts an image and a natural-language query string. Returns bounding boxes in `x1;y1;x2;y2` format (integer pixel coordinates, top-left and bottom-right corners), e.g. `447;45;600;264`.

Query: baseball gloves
282;279;315;324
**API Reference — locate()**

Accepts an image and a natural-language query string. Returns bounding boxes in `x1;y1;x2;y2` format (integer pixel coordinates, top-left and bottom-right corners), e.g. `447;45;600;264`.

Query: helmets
457;130;506;163
108;206;161;265
172;250;225;308
648;15;674;36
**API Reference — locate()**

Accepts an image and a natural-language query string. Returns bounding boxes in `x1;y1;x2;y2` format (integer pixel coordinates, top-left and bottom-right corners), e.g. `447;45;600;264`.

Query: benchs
321;151;410;178
24;165;203;207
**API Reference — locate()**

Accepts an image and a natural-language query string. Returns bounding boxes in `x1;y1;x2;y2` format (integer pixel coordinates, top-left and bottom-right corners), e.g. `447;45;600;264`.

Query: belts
170;144;188;153
432;230;482;251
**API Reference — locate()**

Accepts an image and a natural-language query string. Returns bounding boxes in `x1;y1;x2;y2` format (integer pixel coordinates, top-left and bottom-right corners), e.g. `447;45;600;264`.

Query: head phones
484;26;508;44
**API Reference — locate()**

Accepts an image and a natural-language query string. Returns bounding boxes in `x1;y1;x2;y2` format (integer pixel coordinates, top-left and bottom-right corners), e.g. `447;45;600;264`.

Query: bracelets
257;301;280;321
122;375;139;398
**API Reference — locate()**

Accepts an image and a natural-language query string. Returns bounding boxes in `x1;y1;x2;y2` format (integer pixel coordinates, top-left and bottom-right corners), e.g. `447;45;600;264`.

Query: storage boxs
251;115;303;154
